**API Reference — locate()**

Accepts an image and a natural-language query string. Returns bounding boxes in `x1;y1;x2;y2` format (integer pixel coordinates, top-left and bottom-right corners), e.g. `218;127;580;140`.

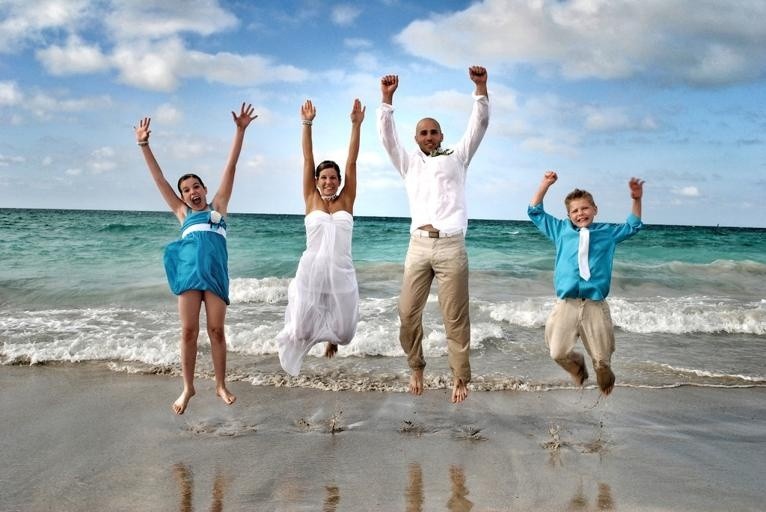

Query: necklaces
321;194;336;201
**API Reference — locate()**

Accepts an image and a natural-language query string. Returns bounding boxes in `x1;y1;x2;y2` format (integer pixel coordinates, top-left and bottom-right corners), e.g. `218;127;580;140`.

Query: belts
414;229;463;239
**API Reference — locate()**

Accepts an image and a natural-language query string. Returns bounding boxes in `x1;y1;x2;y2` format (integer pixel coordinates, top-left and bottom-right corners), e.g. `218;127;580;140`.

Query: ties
578;227;592;282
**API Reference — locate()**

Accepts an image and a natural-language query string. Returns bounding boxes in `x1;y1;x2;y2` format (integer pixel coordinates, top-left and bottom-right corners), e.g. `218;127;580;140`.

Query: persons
379;65;492;404
276;99;366;377
133;101;258;416
527;170;646;396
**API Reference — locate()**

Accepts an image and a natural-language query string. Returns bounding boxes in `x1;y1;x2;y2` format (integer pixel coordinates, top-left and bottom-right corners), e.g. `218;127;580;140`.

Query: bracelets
301;119;312;125
137;141;148;145
